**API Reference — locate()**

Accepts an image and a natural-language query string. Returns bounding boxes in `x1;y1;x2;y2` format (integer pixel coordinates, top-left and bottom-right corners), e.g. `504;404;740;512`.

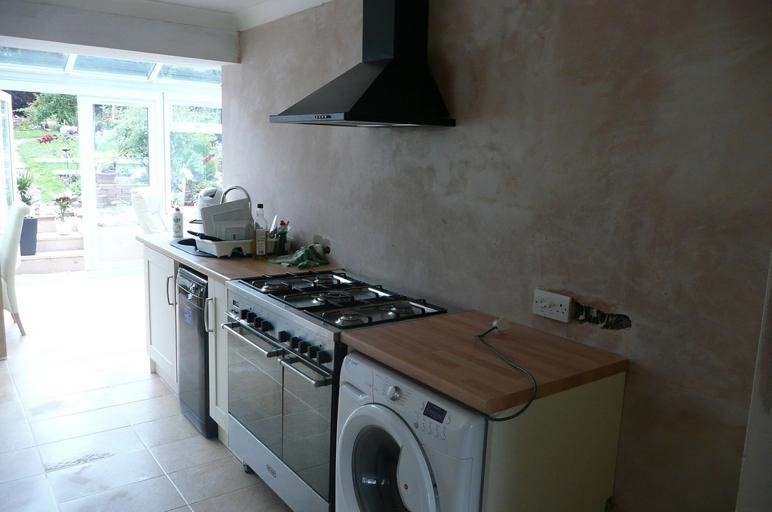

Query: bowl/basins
265;234;294;256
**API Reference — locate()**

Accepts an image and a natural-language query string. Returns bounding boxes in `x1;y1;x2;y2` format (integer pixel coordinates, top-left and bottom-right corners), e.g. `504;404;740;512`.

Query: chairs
0;203;32;338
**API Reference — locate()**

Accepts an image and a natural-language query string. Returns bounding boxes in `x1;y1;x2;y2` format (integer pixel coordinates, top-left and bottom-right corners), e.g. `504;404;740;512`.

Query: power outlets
529;288;574;324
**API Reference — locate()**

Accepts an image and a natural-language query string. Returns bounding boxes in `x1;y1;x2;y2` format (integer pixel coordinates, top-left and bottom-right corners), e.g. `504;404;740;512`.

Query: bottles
172;207;183;238
253;203;268;260
276;219;288;256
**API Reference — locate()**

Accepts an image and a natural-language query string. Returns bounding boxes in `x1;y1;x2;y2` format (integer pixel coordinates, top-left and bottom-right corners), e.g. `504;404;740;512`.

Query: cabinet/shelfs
137;238;176;392
205;278;230;435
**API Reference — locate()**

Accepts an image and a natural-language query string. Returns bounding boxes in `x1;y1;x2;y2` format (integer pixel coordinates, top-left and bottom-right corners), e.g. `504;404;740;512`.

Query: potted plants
17;171;40;256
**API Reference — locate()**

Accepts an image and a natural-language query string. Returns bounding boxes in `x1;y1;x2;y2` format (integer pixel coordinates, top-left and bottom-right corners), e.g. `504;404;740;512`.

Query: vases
56;218;73;236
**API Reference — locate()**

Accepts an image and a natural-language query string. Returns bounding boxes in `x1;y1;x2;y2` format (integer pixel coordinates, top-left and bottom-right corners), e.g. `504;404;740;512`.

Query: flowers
50;190;79;220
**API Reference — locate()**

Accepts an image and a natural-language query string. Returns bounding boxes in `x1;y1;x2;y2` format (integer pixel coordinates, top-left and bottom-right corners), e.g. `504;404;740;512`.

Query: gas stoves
223;270;449;375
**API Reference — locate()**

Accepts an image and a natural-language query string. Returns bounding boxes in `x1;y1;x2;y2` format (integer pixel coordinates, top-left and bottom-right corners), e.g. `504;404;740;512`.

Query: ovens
220;316;347;512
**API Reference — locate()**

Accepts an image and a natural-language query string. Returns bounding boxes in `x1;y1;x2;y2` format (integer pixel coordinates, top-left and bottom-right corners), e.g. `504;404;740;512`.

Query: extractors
269;0;456;128
